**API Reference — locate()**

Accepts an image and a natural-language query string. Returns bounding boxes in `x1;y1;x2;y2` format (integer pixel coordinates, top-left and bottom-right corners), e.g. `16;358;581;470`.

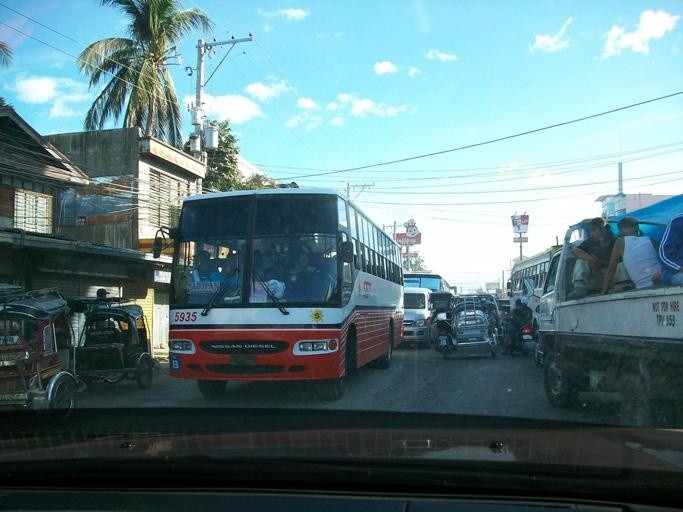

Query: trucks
536;218;683;408
402;273;451;348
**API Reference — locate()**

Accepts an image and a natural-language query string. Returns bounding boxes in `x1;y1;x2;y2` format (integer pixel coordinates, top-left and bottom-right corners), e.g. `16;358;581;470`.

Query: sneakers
567;288;586;300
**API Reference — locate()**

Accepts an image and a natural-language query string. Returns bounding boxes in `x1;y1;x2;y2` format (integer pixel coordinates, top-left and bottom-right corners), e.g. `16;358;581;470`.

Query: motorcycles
431;320;535;356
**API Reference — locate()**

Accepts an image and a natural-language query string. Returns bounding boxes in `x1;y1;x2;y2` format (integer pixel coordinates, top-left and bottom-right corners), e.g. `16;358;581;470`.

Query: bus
509;240;563;314
151;182;407;402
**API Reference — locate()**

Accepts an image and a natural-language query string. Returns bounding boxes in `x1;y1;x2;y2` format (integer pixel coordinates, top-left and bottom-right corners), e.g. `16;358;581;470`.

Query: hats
97;288;110;296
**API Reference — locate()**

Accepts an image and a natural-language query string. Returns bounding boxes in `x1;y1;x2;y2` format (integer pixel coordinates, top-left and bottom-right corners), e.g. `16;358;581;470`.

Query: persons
192;251;210;281
430;307;452;328
97;288;110;308
291;253;320;281
500;299;532;355
568;214;683;298
